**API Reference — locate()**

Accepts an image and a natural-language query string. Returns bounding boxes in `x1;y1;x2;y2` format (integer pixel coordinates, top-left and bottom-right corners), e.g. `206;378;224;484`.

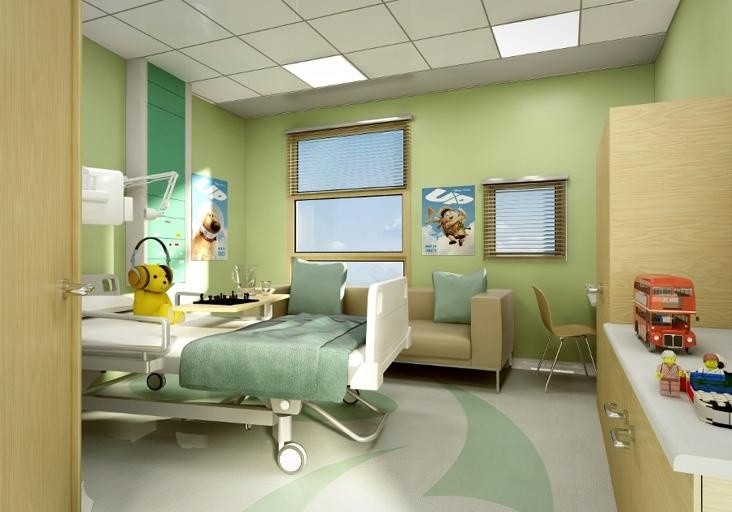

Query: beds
78;274;414;473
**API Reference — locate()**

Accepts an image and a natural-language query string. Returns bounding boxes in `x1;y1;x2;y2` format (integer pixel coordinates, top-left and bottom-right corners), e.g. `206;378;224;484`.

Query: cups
232;264;258;296
260;281;271;296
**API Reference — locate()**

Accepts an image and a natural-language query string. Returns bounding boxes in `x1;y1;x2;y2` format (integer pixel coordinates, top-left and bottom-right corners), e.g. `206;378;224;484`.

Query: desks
600;322;732;512
175;291;290;322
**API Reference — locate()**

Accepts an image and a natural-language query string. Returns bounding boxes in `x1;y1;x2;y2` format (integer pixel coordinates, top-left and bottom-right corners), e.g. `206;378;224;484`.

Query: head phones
128;236;173;289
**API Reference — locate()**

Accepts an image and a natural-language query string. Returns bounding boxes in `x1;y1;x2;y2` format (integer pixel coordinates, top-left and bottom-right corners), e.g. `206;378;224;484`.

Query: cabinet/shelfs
595;97;732;422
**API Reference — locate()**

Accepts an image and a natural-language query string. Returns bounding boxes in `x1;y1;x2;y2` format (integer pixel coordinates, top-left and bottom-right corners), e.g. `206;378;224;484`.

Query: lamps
123;170;179;219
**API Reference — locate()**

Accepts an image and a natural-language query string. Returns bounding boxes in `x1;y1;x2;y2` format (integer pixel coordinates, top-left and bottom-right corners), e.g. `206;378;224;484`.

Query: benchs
265;283;512;392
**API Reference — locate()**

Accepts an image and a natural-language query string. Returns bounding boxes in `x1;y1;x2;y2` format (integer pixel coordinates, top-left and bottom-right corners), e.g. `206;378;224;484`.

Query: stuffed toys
129;262;187;325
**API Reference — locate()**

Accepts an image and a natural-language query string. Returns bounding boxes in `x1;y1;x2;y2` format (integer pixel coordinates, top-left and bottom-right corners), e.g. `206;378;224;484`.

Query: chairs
531;285;596;391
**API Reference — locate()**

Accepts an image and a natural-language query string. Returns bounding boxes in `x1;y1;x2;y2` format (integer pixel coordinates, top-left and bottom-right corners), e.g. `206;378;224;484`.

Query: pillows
432;268;487;323
288;257;347;315
